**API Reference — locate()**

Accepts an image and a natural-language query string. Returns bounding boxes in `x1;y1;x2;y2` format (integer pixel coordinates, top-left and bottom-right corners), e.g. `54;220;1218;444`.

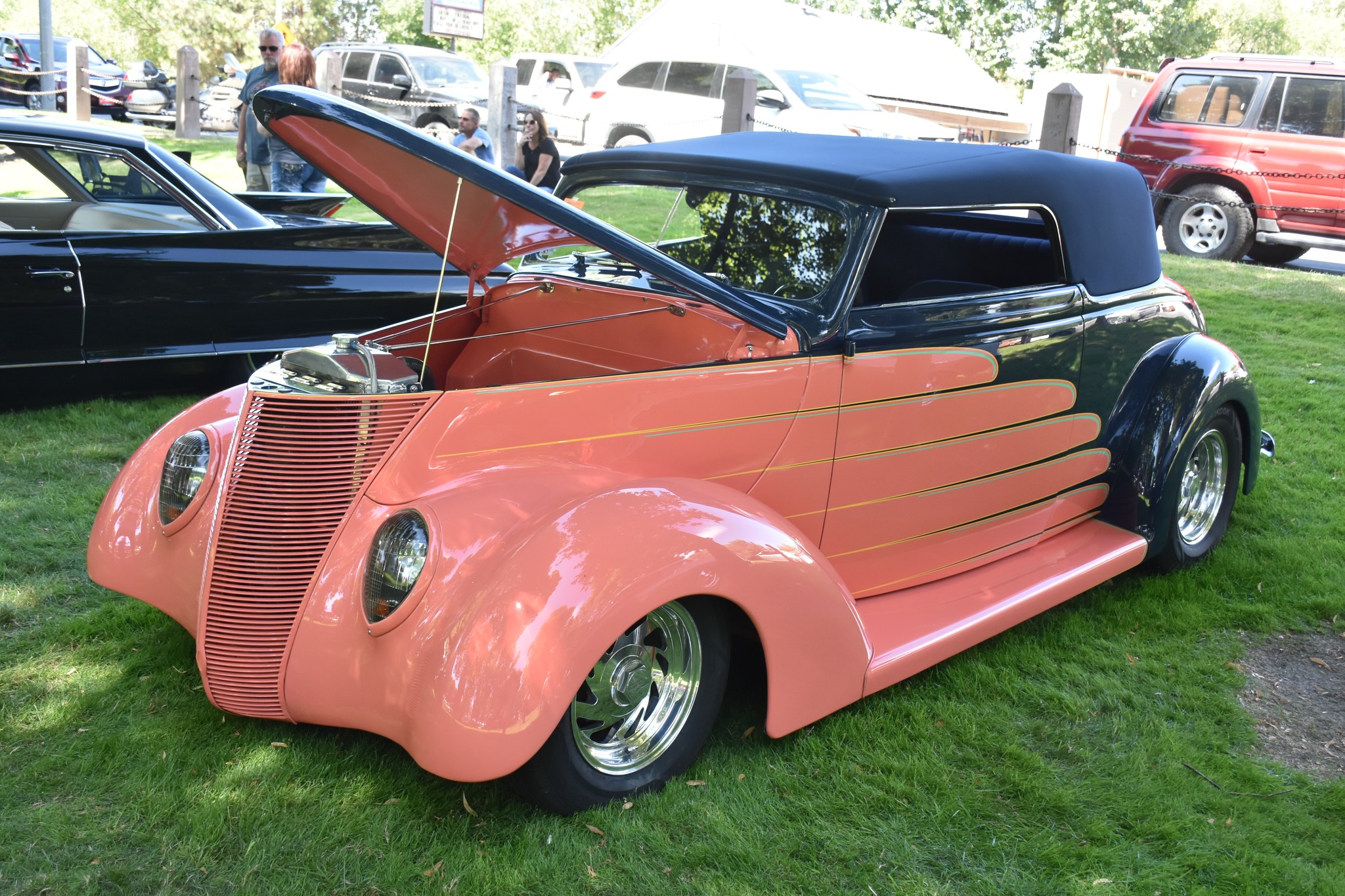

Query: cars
0;34;134;124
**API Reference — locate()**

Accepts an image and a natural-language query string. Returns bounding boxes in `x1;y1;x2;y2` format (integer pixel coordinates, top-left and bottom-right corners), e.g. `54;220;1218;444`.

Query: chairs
899;279;999;298
77;154;154;196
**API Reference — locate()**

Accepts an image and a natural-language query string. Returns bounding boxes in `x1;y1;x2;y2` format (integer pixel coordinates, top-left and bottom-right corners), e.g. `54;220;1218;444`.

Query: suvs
1114;51;1345;263
309;40;533;148
580;60;959;155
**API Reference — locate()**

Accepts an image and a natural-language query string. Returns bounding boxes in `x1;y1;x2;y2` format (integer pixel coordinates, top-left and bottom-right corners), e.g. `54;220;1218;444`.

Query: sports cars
0;116;517;401
84;83;1277;822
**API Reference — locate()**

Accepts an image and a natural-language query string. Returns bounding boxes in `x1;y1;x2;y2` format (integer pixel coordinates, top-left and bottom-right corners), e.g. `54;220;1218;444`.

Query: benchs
60;204;210;228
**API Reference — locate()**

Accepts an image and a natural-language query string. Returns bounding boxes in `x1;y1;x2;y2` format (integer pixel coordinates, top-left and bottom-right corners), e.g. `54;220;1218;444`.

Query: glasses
259;46;281;52
523;120;537;124
458;117;469;121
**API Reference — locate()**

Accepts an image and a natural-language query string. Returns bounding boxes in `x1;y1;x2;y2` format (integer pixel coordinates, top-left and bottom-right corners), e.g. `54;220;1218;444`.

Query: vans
507;50;616;145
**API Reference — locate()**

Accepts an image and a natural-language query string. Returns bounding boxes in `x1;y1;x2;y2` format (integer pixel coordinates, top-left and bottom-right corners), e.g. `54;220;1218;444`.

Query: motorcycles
123;52;249;132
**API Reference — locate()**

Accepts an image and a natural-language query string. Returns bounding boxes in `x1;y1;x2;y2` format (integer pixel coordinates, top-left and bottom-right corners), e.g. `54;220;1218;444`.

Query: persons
540;67;570;105
236;27;286;191
448;108;495;165
249;44;329;193
503;111;562;196
